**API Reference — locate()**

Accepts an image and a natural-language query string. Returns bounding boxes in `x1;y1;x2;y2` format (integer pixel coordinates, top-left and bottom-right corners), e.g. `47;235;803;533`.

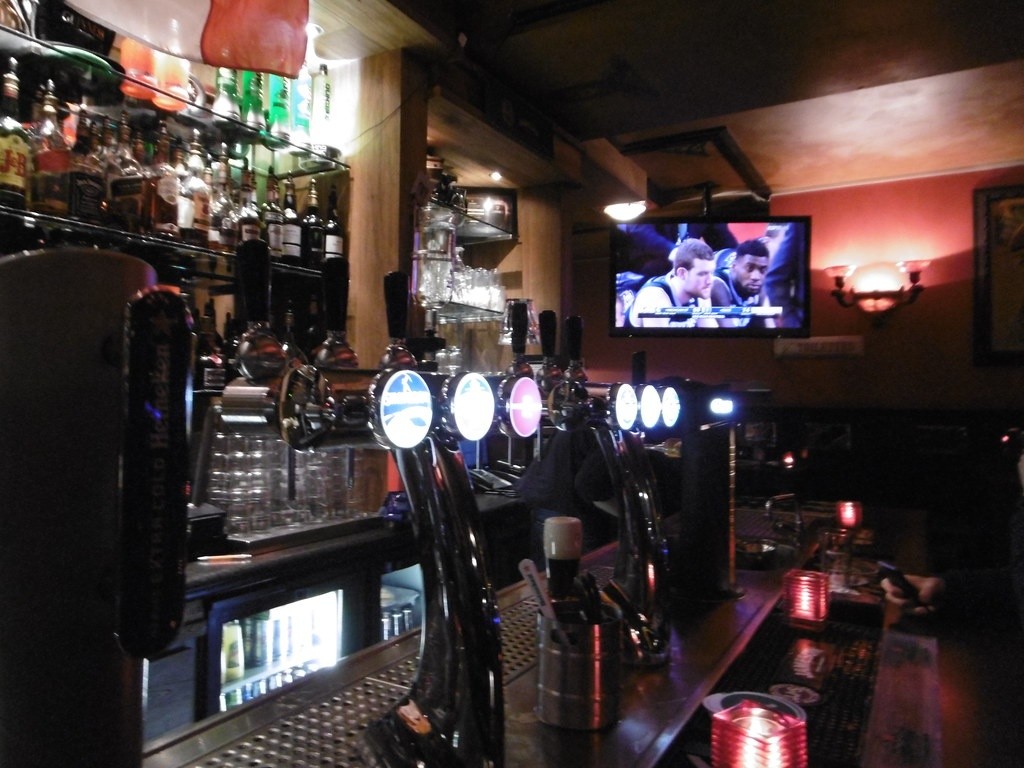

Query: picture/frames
972;184;1023;368
452;185;517;242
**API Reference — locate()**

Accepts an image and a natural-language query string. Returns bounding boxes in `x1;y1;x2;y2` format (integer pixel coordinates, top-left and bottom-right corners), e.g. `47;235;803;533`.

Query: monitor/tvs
607;214;813;339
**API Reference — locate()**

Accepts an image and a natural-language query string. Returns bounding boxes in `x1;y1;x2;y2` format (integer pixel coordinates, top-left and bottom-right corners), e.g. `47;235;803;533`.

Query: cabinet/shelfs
1;0;592;748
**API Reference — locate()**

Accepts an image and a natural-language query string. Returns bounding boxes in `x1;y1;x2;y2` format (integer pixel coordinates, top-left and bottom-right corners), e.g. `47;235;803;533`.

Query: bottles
119;8;333;171
0;72;345;266
304;293;327;348
282;299;309;366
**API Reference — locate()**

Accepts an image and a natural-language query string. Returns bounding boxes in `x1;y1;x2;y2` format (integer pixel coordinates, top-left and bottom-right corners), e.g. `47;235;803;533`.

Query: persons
881;457;1024;628
531;426;612;567
615;226;802;328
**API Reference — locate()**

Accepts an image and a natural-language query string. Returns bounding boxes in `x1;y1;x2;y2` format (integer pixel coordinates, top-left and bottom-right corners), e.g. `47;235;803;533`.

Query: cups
537;601;622;731
543;515;582;601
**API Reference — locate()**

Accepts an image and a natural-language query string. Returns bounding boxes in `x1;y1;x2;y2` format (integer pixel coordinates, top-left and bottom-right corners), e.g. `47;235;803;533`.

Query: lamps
831;270;925;325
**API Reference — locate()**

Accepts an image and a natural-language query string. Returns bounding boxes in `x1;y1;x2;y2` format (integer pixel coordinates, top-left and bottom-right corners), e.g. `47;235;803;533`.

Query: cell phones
876;561;921;606
